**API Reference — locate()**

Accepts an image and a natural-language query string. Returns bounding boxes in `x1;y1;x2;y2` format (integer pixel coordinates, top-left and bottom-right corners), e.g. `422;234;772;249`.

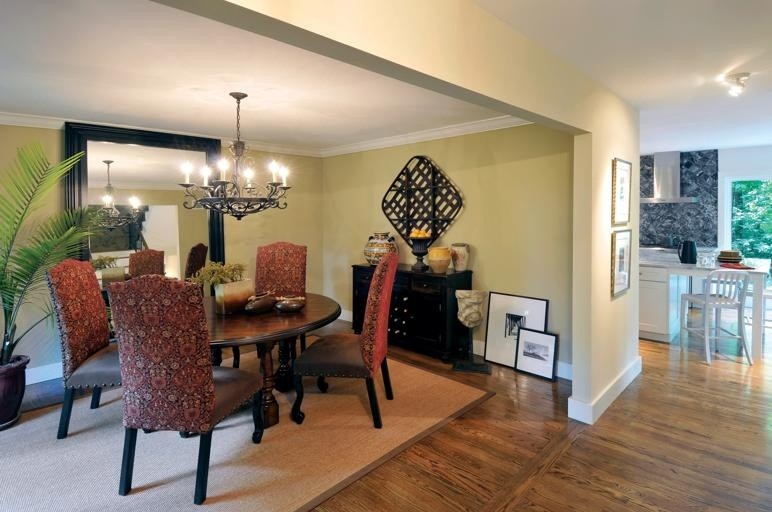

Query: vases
424;246;455;277
360;230;400;270
447;243;471;273
403;234;435;272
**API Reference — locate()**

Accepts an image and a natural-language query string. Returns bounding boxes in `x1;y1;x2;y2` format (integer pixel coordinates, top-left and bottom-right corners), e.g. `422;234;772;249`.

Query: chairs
125;248;167;279
100;271;268;507
227;239;310;371
289;249;400;431
39;254;124;443
180;240;209;279
679;266;754;366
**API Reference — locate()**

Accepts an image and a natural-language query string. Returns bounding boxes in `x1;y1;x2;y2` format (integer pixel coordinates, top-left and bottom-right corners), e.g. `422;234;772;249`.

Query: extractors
639;151;699;204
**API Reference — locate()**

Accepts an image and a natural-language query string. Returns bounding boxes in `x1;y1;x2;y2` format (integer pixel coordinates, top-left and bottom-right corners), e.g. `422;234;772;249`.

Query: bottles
364;232;398;266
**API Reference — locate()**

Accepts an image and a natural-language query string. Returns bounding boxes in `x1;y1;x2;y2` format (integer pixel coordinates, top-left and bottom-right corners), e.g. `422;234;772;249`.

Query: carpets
0;330;500;509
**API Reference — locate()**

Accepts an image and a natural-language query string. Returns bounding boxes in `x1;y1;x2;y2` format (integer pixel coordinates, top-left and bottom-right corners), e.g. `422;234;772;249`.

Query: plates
717;255;744;263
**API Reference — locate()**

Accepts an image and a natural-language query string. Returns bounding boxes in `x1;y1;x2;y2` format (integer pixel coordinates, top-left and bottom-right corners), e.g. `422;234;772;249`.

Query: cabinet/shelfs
344;255;478;368
638;261;690;346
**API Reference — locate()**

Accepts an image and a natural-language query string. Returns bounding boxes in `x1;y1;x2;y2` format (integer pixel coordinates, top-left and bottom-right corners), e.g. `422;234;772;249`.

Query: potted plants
0;130;113;440
185;254;257;319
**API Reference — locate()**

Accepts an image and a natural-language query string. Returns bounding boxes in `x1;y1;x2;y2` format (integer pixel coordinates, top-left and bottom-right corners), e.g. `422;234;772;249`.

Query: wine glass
407;235;431;272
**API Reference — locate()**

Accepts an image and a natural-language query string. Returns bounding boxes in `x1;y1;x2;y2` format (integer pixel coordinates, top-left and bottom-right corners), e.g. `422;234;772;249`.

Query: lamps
177;90;293;223
724;70;753;98
88;158;146;236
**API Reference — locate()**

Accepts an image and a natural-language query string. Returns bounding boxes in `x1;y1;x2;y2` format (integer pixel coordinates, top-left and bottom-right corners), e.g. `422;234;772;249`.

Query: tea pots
677;240;697;264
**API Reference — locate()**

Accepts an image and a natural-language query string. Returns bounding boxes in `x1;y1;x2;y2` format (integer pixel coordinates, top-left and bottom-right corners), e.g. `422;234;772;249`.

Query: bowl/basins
719;251;740;257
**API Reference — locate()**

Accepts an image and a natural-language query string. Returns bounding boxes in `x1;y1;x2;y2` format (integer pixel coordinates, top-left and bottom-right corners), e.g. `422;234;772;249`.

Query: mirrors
59;120;226;341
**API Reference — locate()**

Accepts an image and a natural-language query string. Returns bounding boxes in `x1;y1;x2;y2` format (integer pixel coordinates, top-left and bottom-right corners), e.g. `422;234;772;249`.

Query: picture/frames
607;226;633;299
512;323;560;383
482;289;550;369
611;155;633;227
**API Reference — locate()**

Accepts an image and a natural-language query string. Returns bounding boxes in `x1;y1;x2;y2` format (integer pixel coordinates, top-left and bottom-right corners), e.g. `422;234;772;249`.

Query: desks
676;254;771;366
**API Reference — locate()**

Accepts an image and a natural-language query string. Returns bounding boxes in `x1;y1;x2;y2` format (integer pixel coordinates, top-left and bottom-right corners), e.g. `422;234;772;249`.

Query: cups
695;252;716;268
428;247;451;274
451;242;471;273
